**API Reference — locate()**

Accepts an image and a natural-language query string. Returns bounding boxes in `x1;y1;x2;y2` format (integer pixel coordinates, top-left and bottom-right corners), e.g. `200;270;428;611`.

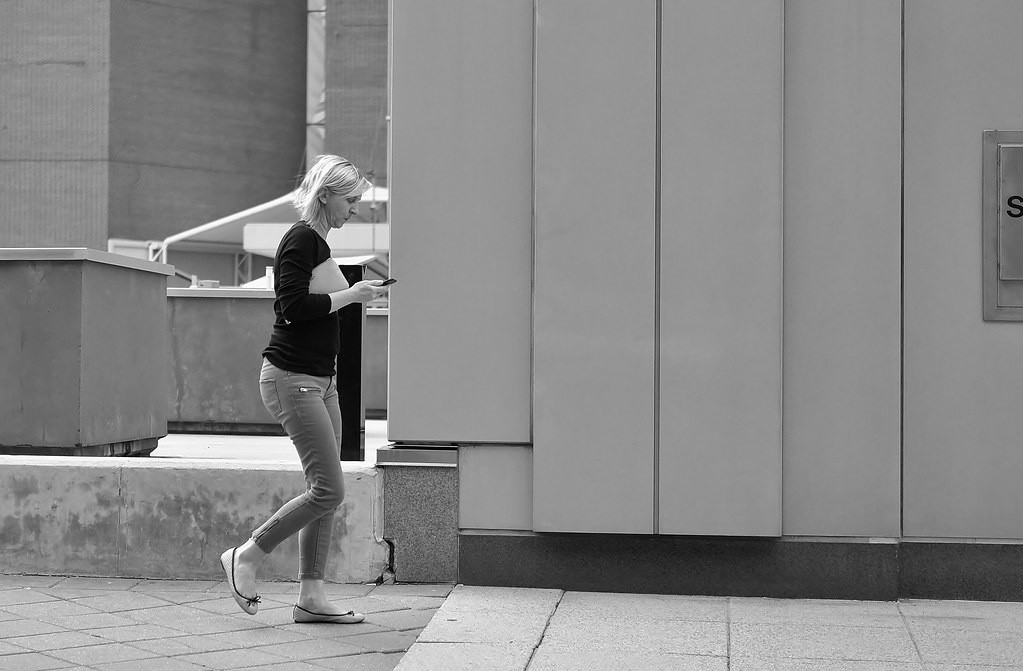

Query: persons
219;153;387;622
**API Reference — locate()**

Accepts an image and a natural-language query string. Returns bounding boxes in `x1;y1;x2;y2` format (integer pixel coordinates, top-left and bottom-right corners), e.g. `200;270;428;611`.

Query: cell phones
380;278;397;287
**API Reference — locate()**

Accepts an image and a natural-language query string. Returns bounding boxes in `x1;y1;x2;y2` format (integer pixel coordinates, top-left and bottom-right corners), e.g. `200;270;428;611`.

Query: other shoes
219;546;259;615
292;603;365;624
